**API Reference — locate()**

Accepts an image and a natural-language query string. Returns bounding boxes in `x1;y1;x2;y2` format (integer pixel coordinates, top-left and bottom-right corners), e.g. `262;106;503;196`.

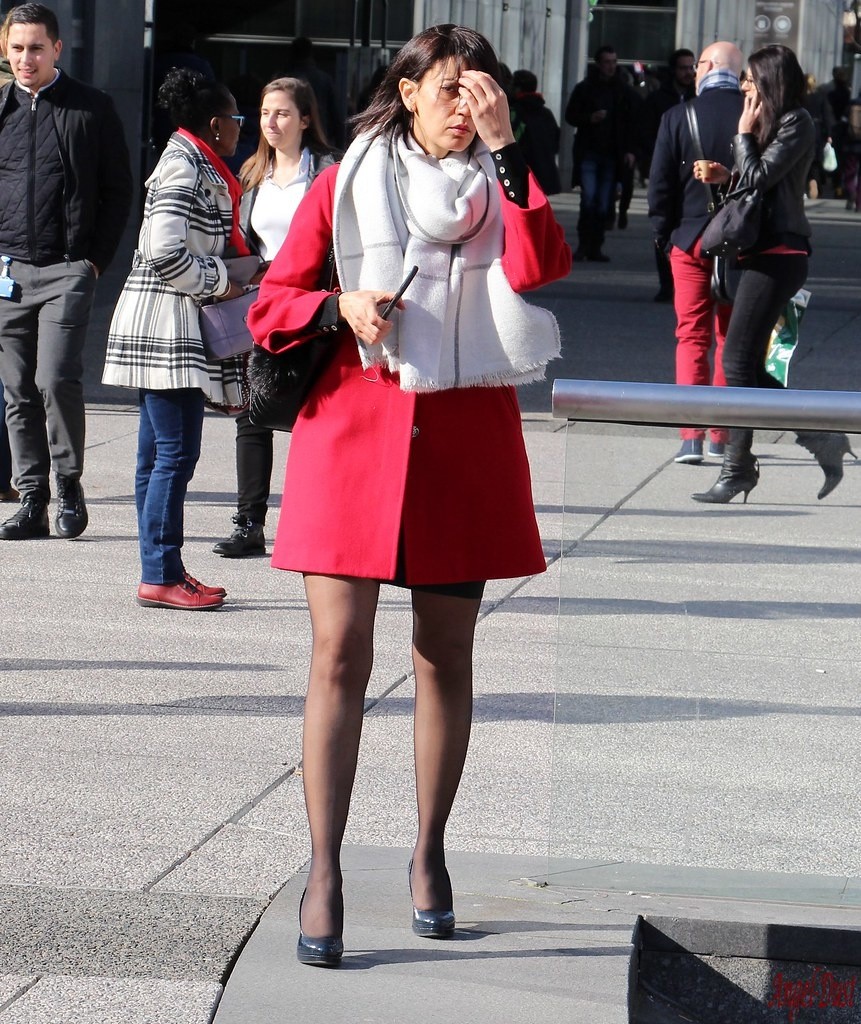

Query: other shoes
809;179;818;199
708;440;725;456
673;437;704;463
586;250;610;262
571;245;587;263
651;288;674;303
617;211;628;230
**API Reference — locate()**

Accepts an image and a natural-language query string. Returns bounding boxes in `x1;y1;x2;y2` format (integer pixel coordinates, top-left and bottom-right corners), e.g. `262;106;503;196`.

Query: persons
212;77;344;555
644;40;746;463
0;3;134;541
100;66;250;611
0;6;16;88
690;44;858;504
564;46;861;264
246;23;573;965
513;70;562;197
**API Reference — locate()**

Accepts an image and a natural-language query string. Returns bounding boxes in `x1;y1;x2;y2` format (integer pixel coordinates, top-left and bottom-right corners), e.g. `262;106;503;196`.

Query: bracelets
219;280;232;297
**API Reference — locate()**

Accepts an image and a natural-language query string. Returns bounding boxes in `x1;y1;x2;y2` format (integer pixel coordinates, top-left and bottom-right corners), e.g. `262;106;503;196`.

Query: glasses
693;59;718;72
745;77;756;86
218;115;245;127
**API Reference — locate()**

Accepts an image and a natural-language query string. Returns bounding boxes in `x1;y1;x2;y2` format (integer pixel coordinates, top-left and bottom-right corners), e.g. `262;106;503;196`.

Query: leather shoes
135;577;223;609
178;570;226;597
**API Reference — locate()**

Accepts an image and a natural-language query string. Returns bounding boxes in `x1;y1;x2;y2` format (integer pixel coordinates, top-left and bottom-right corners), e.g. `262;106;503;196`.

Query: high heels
408;859;455;936
297;885;344;964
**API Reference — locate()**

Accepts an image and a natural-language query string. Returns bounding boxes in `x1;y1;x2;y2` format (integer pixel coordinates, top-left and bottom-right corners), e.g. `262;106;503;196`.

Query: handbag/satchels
700;157;770;257
247;246;338;433
710;249;744;305
199;254;271;363
822;141;838;172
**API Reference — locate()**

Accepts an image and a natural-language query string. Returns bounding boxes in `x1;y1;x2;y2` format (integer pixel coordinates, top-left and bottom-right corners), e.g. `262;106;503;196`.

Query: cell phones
381;265;418;320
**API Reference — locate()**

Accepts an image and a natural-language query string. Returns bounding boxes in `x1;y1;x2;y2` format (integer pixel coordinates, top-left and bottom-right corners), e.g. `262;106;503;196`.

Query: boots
212;514;266;554
796;428;858;500
55;474;88;538
0;494;51;540
690;443;761;504
